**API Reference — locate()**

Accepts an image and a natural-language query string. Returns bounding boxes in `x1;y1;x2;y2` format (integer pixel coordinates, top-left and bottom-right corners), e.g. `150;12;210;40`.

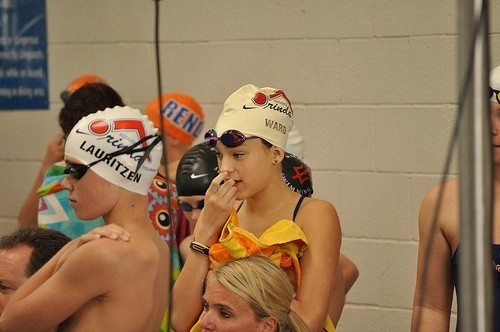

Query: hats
65;106;163;195
487;65;500;103
214;84;294;152
175;141;224;196
61;74;106;102
282;151;313;198
59;82;126;134
146;93;204;147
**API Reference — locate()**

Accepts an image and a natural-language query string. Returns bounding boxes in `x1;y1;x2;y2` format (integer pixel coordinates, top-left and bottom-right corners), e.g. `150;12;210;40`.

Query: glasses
204;129;248;147
62;165;88;180
177;199;205;212
488;86;500;104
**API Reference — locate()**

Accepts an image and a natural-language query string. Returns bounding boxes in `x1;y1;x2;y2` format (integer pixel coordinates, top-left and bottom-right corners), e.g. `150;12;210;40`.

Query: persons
0;73;360;332
410;65;500;332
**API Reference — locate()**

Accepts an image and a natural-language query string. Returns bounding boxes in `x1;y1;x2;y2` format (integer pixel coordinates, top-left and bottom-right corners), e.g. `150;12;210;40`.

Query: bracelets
189;241;212;255
